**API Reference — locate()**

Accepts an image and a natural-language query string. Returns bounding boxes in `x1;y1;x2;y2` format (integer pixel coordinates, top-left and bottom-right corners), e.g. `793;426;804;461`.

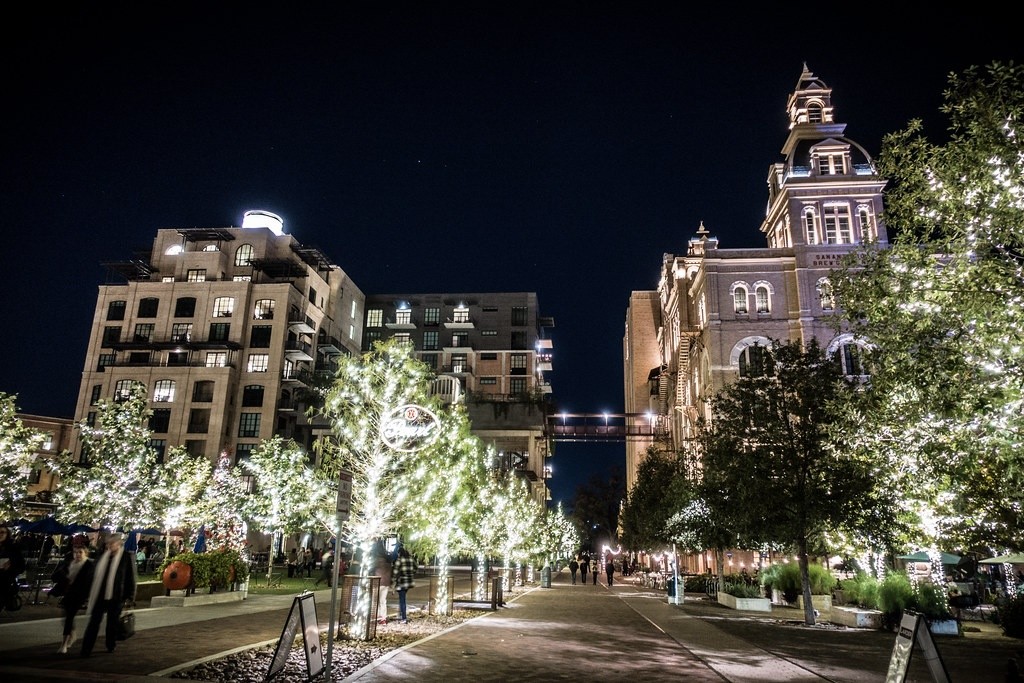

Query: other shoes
80;651;90;657
57;640;71;653
108;647;114;651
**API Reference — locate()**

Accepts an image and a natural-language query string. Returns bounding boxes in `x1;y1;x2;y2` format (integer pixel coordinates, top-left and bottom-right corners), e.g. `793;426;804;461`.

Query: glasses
0;531;7;534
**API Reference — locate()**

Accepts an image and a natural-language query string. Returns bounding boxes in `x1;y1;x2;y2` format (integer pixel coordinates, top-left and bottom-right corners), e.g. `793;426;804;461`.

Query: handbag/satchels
47;595;64;607
117;605;135;641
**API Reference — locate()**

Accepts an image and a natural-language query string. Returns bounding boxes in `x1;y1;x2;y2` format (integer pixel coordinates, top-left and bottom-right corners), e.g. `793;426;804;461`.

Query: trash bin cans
667;578;684;605
541;567;552;589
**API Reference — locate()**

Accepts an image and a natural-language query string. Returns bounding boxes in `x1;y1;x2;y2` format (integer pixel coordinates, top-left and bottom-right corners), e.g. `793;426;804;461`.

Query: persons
390;548;418;623
569;557;579;585
605;559;615;586
580;558;587;584
580;550;636;576
288;547;346;587
0;524;186;656
592;558;599;585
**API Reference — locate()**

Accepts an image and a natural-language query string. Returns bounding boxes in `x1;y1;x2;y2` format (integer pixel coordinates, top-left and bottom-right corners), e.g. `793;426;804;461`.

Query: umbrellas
0;517;213;568
897;548;1024;565
